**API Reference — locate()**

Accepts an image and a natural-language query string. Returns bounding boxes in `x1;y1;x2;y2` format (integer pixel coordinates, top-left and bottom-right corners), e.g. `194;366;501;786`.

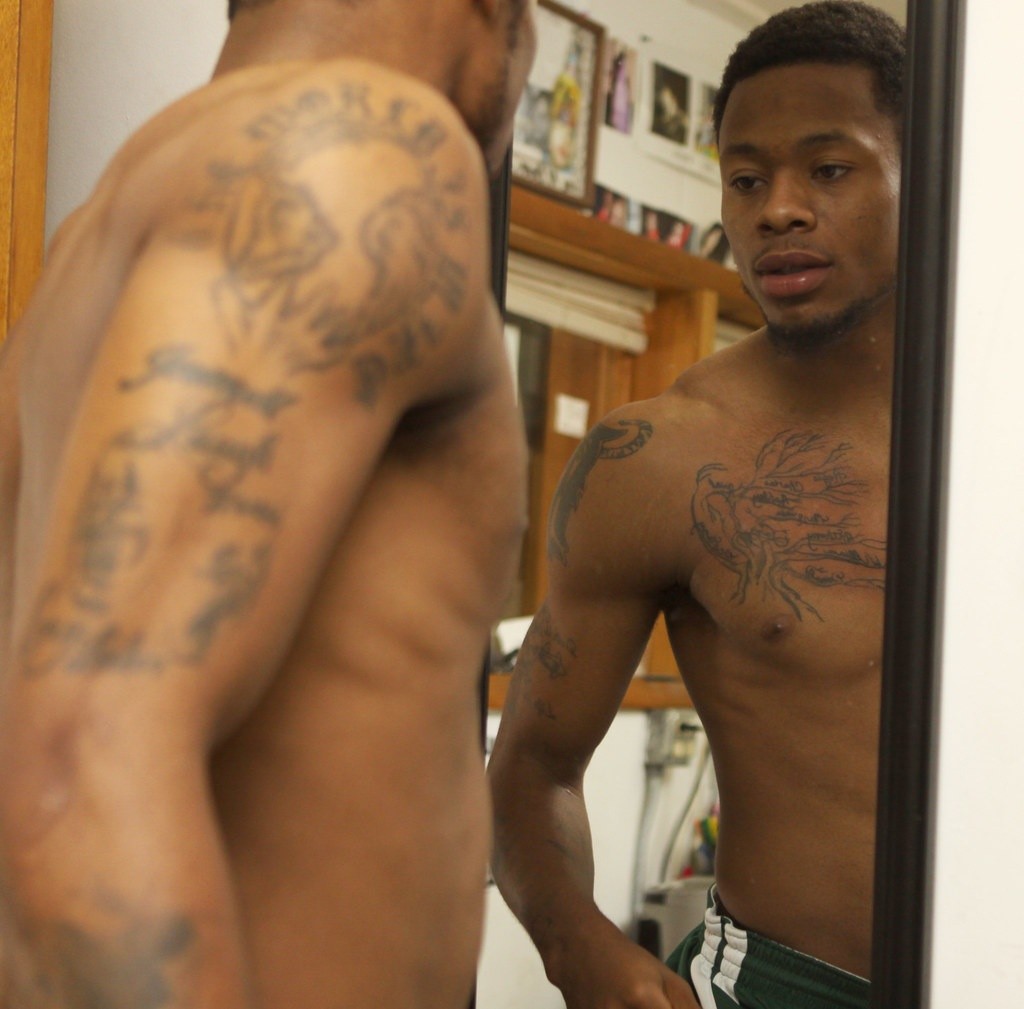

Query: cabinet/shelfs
481;181;774;722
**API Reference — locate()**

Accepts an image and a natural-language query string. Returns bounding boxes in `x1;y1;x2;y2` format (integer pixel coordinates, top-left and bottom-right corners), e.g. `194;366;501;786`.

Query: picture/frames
510;0;612;210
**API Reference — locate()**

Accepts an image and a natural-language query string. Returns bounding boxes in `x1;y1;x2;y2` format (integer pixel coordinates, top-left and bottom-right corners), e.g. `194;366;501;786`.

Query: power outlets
642;707;699;765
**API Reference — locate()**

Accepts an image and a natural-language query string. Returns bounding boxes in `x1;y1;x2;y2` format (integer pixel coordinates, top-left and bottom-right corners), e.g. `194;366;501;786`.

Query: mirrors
427;1;963;1005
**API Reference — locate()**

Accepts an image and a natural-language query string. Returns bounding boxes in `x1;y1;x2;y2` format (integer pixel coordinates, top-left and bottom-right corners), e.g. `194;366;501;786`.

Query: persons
0;0;540;1009
485;1;927;1008
